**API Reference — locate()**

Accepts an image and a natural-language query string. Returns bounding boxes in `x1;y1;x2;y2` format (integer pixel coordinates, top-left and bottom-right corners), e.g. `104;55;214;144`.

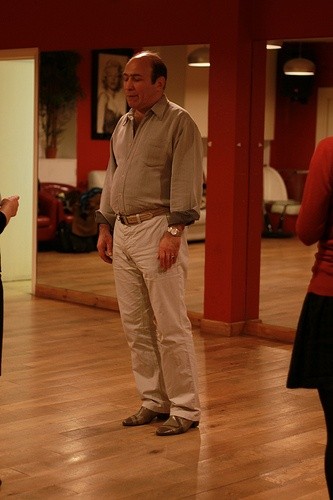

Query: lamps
282;41;316;77
186;44;210;67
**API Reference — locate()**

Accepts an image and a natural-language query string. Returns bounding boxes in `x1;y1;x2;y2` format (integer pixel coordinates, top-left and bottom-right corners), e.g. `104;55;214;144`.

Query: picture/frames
90;48;133;140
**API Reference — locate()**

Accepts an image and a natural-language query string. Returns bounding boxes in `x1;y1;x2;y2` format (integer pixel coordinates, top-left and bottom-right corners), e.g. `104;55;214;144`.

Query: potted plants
38;51;85;159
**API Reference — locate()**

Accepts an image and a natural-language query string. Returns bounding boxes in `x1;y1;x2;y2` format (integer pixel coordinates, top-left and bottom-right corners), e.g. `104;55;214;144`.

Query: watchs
167;227;183;237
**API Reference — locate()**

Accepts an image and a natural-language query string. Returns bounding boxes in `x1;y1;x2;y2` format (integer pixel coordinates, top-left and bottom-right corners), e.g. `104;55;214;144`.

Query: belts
115;207;170;226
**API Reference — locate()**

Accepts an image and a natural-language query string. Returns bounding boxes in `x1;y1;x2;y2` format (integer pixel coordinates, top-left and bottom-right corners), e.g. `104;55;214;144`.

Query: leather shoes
154;415;199;436
122;405;170;426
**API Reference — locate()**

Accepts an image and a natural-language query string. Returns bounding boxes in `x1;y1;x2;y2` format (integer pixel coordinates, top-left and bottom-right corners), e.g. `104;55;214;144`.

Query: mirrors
33;44;212;315
258;37;332;329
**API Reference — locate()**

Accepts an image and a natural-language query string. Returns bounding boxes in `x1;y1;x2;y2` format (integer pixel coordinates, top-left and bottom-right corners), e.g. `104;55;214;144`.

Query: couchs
36;188;58;240
88;168;206;242
40;182;80;223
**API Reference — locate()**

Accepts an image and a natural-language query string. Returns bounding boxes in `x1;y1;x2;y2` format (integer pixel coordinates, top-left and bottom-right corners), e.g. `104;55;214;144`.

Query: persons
96;49;206;436
285;137;333;500
0;195;21;378
